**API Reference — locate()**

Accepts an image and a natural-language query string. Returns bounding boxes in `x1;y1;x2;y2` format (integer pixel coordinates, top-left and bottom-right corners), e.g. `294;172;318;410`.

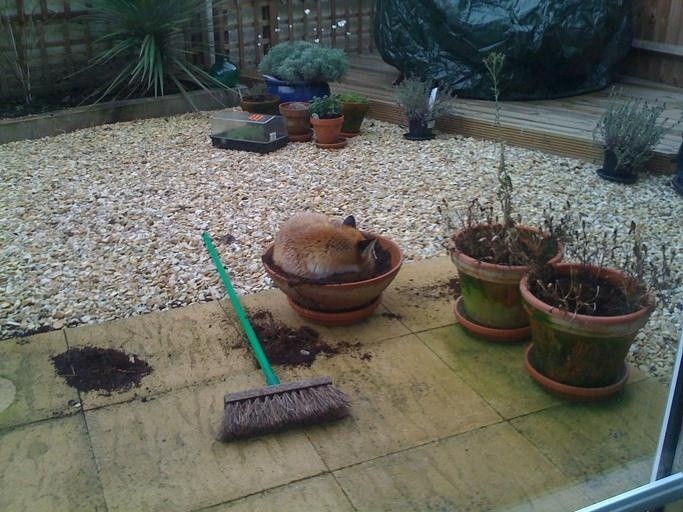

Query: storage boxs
209;111;289;154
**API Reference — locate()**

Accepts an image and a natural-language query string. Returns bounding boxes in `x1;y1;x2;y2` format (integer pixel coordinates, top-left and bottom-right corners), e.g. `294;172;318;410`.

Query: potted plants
241;40;370;143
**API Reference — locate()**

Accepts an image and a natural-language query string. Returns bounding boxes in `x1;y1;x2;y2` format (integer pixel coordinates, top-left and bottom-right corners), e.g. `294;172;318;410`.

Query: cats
269;210;379;280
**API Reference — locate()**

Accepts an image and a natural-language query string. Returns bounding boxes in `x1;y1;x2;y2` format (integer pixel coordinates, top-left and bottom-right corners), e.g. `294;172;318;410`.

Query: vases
519;265;657;385
262;230;405;312
208;48;240;89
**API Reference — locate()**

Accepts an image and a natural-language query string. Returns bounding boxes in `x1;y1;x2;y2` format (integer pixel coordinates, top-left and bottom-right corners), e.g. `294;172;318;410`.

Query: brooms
201;230;351;442
